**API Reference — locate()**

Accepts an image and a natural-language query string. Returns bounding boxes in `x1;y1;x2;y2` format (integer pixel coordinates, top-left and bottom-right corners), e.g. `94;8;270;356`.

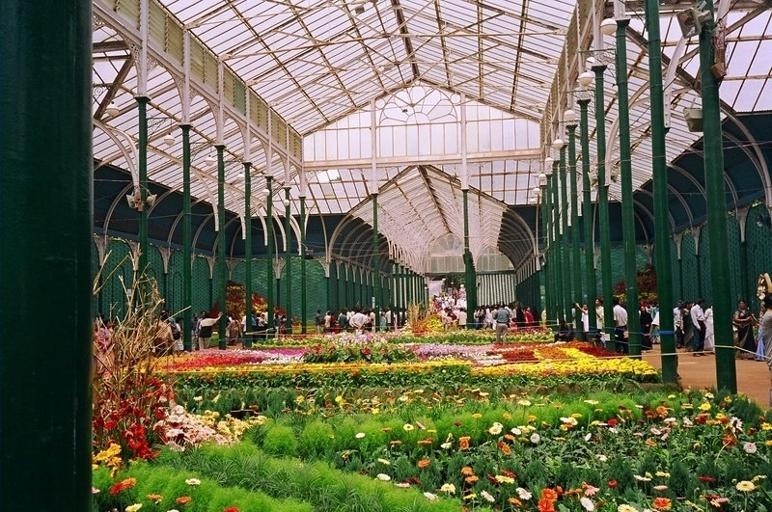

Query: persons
314;304;408;334
95;306;301;371
733;292;771;372
638;296;715;357
436;299;540;345
540;295;628;354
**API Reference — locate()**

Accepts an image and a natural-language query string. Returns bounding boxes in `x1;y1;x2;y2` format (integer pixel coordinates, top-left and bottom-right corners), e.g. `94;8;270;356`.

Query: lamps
528;138;565;206
261;188;271;197
104;101;121;118
205;156;216;167
576;69;595;87
164;134;175;146
282;198;290;207
560;102;579;123
237;173;246;182
598;18;619;37
